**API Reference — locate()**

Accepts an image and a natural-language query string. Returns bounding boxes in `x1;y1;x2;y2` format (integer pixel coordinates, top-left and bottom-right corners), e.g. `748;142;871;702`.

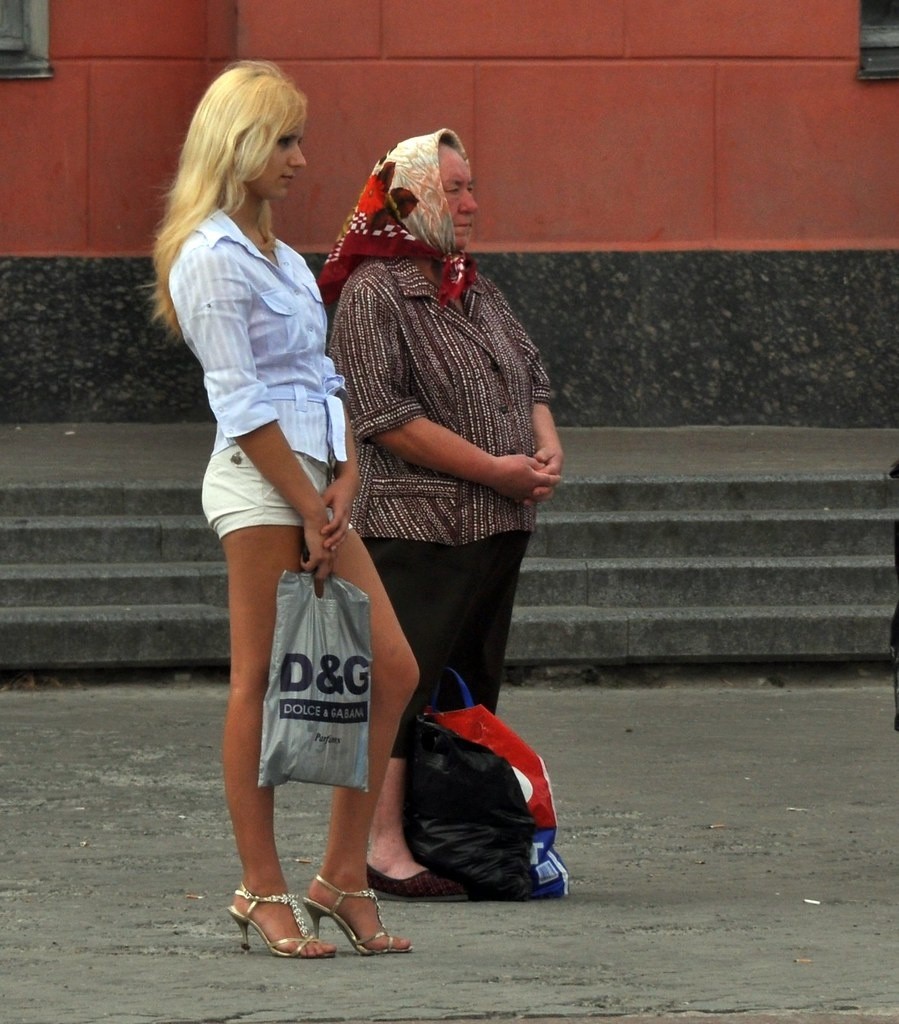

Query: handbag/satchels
257;573;373;795
401;668;569;903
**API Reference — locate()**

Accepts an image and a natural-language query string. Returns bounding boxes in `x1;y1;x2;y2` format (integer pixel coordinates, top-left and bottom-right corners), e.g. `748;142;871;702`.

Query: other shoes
366;858;468;902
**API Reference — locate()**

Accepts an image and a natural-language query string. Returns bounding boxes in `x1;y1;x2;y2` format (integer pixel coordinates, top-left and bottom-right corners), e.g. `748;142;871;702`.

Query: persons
141;58;409;956
316;129;566;902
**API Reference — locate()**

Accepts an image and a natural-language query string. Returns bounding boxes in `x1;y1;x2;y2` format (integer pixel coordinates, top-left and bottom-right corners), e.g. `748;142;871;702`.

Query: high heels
226;880;336;959
303;874;414;955
